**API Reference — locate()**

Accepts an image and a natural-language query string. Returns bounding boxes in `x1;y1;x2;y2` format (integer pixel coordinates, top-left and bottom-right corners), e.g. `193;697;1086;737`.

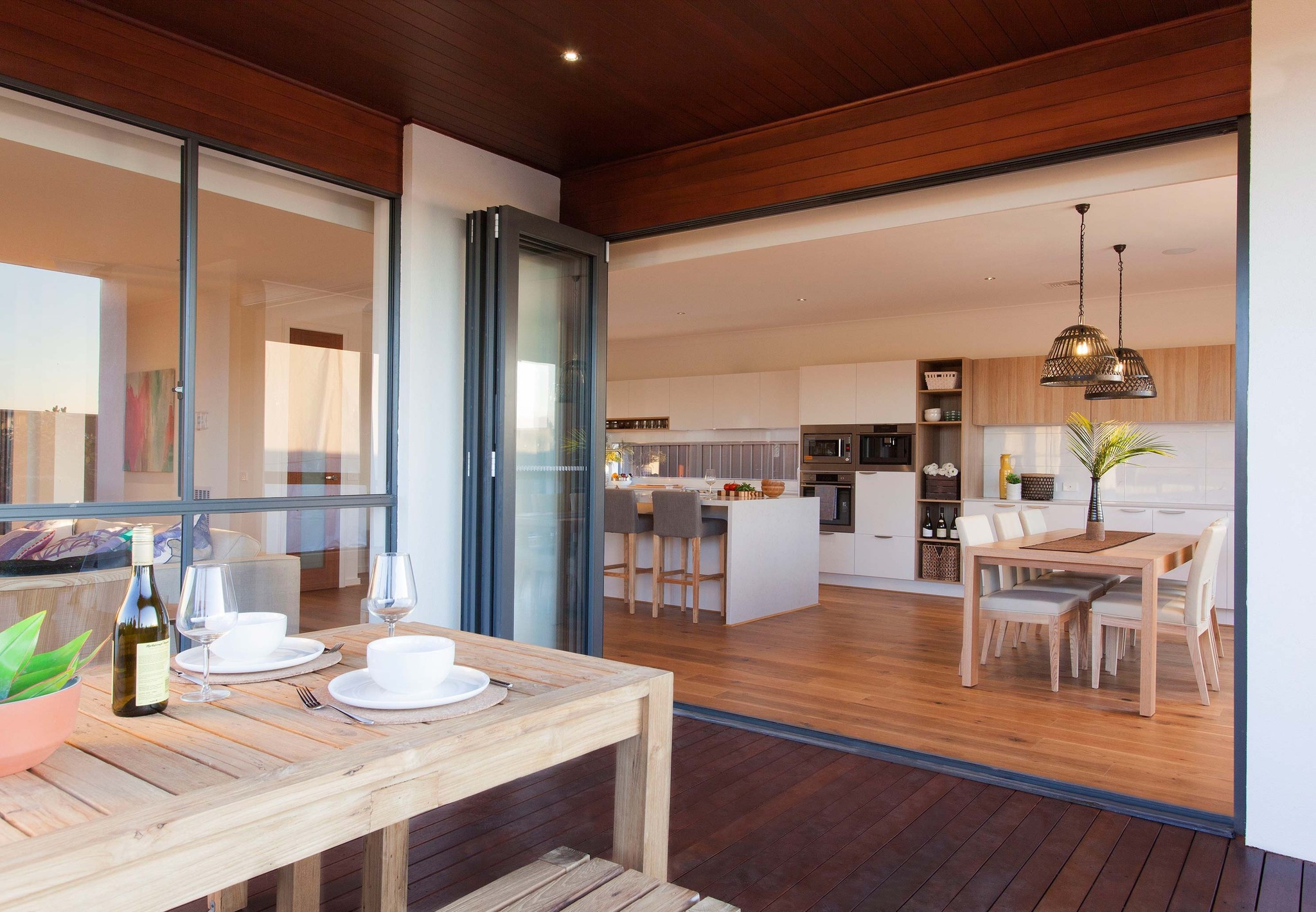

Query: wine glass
367;552;418;637
176;562;239;702
705;469;716;496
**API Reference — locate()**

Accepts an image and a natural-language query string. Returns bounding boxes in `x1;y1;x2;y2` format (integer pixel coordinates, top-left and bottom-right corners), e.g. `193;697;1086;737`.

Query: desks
0;623;675;912
962;528;1201;719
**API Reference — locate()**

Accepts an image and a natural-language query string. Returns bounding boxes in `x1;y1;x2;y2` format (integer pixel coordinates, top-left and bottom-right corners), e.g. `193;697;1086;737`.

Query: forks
296;686;374;725
324;642;344;655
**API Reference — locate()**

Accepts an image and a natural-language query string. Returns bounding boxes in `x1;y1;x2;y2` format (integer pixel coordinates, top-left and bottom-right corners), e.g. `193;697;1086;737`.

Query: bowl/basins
761;479;785;497
205;611;287;661
925;408;941;421
611;477;632;486
944;411;961;421
366;635;456;696
0;674;82;778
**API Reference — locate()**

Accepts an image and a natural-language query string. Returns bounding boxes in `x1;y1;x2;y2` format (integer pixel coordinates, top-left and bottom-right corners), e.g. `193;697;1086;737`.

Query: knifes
169;666;204;686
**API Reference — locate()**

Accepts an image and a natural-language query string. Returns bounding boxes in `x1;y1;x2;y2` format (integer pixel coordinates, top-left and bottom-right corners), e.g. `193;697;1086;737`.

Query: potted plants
0;610;115;778
1062;412;1178;541
1005;473;1022;500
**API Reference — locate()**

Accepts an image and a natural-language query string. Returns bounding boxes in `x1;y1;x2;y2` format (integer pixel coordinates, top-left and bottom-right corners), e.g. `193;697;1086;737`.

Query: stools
602;486;665;616
651;489;726;625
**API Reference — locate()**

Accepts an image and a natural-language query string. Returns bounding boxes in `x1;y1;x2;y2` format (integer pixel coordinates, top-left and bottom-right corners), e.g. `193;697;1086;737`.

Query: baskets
924;372;962;389
922;543;960;581
761;479;785;486
761;486;785;497
1020;472;1054;500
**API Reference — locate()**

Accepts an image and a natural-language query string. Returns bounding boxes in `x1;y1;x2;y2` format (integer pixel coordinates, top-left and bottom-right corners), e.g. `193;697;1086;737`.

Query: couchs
0;529;301;670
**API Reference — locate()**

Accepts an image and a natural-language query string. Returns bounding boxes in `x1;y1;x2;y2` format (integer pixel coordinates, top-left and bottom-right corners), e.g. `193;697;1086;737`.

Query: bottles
999;453;1012;499
605;420;668;429
112;525;172;717
937;507;947;539
950;508;960;540
922;507;933;539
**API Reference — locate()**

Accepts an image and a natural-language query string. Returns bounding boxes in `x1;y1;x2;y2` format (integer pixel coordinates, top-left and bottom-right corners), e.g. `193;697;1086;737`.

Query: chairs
437;844;741;912
953;508;1230;707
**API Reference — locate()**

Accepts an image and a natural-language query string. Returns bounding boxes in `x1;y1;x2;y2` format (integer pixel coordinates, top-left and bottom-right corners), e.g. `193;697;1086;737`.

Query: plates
176;636;323;673
328;664;490;709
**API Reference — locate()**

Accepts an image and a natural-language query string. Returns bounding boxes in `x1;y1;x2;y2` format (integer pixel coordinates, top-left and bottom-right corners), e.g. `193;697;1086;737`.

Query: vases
999;453;1012;499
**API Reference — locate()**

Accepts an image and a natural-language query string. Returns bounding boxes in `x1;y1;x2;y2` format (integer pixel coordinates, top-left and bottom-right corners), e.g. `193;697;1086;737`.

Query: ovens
862;434;909;464
799;471;855;532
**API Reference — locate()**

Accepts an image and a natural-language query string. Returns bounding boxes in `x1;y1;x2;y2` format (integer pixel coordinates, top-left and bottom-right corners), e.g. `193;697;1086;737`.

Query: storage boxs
925;474;959;500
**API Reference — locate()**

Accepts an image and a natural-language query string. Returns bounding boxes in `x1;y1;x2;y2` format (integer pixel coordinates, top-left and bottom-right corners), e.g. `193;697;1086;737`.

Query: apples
618;473;633;477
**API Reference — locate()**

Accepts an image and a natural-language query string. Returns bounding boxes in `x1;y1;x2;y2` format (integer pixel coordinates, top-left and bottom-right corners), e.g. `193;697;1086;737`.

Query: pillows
0;513;212;565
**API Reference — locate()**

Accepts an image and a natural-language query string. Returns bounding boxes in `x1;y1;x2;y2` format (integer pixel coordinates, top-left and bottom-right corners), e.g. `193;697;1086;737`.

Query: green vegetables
736;482;756;491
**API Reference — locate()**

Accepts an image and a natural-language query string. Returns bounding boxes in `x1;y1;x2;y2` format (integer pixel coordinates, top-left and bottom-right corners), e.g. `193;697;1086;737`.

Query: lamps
1040;201;1125;387
1084;245;1157;400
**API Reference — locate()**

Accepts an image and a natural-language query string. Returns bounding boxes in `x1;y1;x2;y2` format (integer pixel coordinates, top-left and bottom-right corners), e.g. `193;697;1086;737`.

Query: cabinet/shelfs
606;359;799;430
915;357;984;585
801;360;917;425
819;472;916;581
972;344;1236;425
964;501;1234;611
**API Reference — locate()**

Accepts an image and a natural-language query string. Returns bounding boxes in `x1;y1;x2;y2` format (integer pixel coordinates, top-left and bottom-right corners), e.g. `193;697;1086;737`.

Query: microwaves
804;434;852;463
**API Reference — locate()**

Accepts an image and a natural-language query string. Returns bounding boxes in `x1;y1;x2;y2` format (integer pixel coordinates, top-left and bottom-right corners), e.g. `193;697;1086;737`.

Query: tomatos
724;483;740;491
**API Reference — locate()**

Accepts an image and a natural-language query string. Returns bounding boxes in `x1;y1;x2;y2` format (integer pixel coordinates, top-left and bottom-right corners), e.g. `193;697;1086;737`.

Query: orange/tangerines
612;473;619;478
